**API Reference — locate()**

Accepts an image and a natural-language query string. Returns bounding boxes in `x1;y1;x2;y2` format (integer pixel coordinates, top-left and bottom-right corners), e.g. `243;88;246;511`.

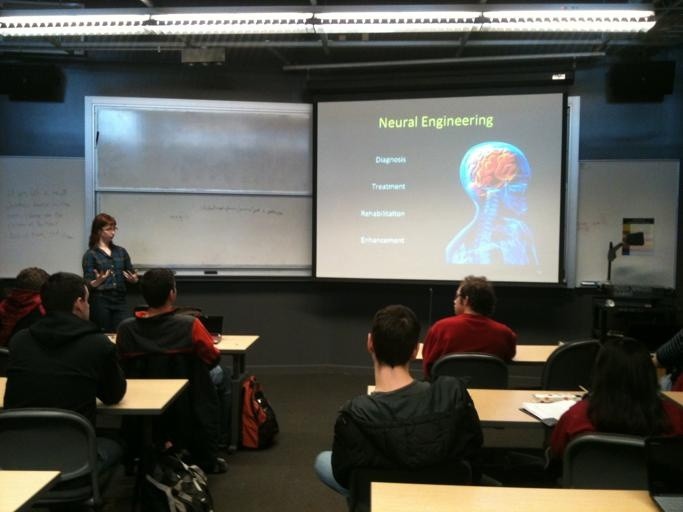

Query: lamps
0;4;657;39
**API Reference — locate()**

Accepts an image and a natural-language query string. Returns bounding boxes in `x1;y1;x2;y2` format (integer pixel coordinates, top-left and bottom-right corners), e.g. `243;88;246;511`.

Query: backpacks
145;455;213;512
241;375;279;449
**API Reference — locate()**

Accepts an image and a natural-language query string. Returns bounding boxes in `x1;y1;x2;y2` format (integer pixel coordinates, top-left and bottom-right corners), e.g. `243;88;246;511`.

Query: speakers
8;62;66;102
605;65;664;103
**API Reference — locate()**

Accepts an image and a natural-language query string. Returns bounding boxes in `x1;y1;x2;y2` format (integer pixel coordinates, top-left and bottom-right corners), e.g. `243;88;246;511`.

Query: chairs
430;354;509;388
563;434;647;490
0;410;116;511
539;338;601;390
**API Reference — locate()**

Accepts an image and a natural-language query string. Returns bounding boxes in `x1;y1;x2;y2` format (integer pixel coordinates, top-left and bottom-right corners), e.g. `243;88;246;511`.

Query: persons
114;268;220;367
547;337;682;463
423;274;517;389
650;327;683;390
81;213;139;332
1;267;51;349
313;304;484;500
1;274;138;511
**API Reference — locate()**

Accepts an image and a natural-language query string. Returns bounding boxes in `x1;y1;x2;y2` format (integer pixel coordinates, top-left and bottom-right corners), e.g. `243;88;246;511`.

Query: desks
0;469;60;511
104;332;262;453
367;384;682;429
414;342;559;366
1;375;191;415
370;481;662;510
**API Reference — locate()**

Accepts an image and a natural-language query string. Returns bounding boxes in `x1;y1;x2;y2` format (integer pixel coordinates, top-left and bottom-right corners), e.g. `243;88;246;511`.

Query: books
520;399;580;429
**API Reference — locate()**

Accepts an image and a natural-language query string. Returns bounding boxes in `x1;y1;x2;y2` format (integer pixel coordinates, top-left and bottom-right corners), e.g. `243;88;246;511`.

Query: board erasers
204;270;219;275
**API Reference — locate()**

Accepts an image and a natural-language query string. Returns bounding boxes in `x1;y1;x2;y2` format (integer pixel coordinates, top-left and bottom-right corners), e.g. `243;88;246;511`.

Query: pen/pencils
577;384;588;394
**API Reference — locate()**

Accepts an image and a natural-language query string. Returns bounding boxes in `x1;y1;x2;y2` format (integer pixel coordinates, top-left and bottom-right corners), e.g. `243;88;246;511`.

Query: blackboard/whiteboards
0;155;84;279
573;156;683;295
91;102;316;271
